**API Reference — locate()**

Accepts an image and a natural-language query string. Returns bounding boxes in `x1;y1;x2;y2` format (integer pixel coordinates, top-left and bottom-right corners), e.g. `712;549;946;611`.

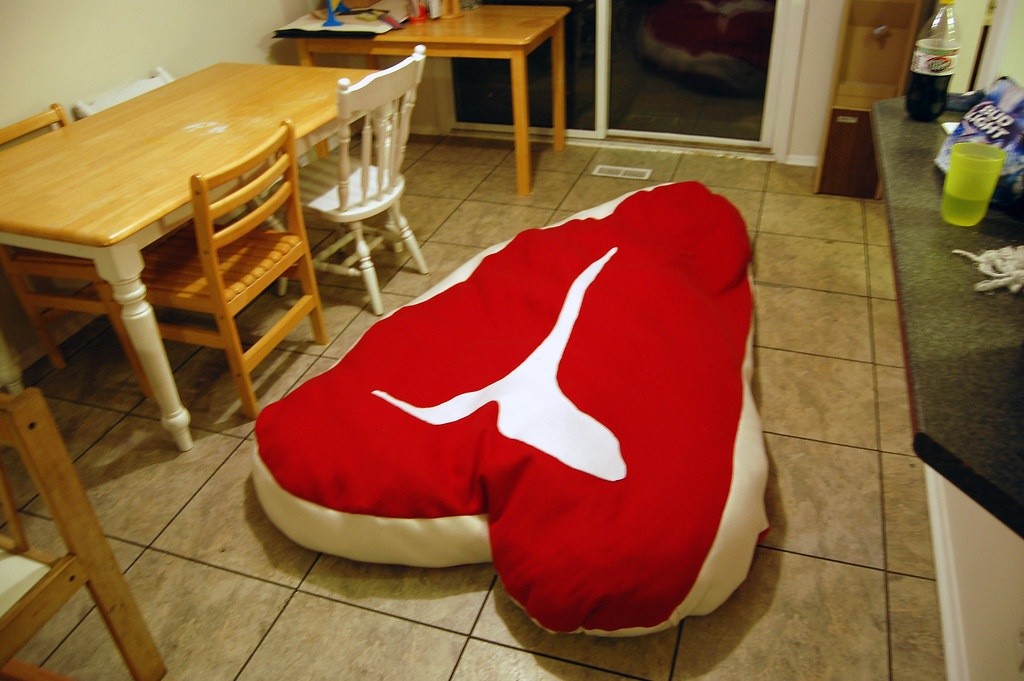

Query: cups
940;142;1007;226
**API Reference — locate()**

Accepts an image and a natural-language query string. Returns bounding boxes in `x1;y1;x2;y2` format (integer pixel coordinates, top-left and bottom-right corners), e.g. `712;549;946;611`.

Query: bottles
905;0;961;121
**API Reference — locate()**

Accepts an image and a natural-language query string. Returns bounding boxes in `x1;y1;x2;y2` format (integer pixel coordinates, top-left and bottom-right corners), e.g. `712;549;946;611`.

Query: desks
0;62;404;452
295;5;571;195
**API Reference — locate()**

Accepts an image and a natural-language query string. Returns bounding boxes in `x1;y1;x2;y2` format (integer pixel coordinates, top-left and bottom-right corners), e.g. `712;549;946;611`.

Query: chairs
0;385;169;681
269;44;428;315
73;67;285;231
0;104;191;398
141;118;329;421
869;93;1024;680
